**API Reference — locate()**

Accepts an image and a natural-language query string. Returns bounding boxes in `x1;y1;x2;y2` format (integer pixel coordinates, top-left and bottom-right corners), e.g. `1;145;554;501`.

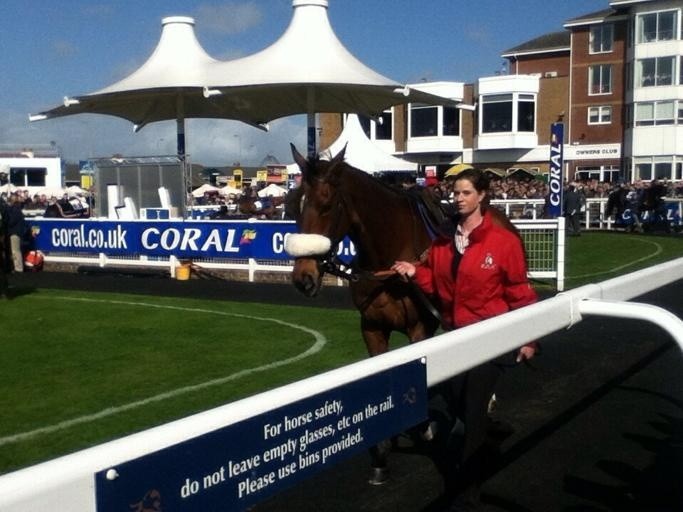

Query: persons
185;182;286;219
1;189;94;296
423;170;438;187
419;176;682;236
390;170;539;464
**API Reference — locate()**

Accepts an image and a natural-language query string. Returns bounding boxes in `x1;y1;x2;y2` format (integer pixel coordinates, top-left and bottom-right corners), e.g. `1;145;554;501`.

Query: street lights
232;134;243;164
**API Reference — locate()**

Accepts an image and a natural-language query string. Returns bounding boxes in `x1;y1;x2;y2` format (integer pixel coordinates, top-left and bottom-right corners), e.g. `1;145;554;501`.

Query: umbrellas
445;162;474;176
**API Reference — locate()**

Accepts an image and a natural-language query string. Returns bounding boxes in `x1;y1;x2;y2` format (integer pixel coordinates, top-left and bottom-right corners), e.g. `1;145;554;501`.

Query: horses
239;195;274;220
42;196;94;219
287;141;528;485
604;183;667;234
